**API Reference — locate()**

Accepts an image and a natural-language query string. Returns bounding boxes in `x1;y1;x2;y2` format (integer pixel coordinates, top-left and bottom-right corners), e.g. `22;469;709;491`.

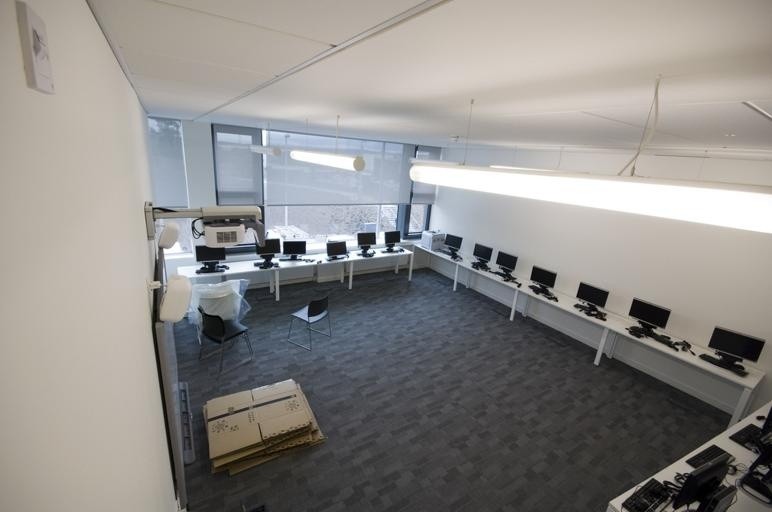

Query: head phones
635;485;661;507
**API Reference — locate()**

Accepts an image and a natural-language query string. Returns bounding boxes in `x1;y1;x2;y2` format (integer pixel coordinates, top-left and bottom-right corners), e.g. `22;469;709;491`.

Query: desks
177;245;414;301
605;402;770;511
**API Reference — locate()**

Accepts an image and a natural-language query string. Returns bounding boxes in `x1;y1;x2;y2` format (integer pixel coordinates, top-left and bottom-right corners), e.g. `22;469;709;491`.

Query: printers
421;231;445;251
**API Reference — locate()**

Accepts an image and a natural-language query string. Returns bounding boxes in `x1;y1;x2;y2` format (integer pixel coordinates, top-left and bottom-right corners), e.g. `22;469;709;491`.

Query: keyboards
622;478;669;512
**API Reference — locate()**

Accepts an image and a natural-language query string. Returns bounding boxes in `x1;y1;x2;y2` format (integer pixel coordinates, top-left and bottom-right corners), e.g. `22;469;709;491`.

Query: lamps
288;116;366;173
250;122;281;156
408;78;771;233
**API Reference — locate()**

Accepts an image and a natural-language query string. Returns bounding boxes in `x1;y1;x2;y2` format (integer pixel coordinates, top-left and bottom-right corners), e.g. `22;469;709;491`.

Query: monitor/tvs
672;407;772;512
195;231;404;273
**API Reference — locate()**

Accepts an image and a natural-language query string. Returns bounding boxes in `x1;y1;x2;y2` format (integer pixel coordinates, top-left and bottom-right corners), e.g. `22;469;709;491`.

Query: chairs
286;298;332;351
197;306;254;375
